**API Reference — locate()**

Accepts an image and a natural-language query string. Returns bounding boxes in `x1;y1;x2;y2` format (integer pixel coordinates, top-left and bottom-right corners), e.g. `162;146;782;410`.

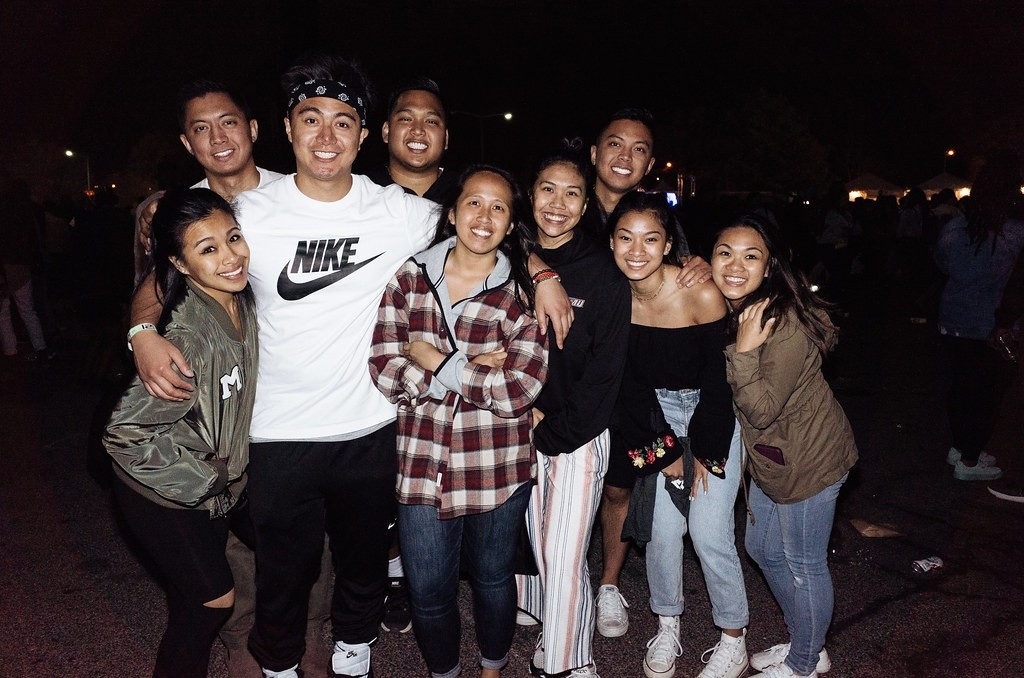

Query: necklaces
629;281;665;301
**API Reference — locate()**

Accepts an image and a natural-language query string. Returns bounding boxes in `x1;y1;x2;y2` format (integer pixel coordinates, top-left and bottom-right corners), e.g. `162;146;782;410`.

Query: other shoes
517;610;538;628
327;635;378;677
987;477;1024;502
261;663;304;678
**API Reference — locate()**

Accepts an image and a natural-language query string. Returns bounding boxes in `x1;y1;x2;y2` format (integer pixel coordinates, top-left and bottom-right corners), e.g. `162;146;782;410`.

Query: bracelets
531;269;557;280
127;322;157;352
532;272;561;289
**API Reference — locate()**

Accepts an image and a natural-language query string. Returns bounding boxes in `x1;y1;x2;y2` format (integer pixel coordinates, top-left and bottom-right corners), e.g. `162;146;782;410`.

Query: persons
881;161;1024;502
0;176;134;362
99;60;857;678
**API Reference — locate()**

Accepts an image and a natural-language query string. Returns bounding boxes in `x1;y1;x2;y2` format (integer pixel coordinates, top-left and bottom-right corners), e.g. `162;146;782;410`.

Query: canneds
910;555;944;574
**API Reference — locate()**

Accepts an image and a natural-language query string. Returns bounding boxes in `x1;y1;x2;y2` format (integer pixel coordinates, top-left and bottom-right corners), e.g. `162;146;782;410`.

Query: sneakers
643;615;684;678
750;642;832;673
955;461;1003;481
527;631;546;678
749;663;818;678
569;662;598;678
594;584;630;638
694;628;749;678
381;576;412;634
945;447;997;466
